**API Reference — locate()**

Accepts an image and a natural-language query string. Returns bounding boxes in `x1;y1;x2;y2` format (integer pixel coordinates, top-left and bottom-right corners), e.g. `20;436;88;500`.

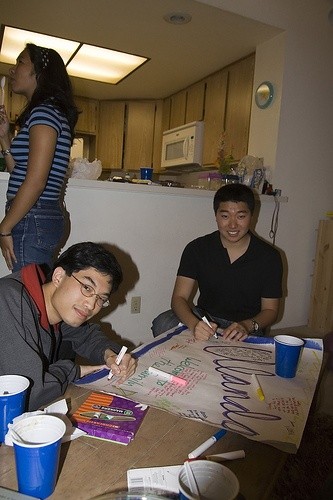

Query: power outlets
130;296;141;313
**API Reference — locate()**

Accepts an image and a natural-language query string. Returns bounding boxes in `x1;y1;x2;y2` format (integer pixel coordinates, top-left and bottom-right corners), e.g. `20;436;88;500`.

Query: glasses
64;268;111;309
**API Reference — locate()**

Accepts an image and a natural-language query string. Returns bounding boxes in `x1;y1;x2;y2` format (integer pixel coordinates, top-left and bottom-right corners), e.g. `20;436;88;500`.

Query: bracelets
0;233;12;236
1;149;11;157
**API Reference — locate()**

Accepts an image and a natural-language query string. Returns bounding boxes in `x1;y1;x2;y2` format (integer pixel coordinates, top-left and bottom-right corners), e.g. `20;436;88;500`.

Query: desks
0;324;329;500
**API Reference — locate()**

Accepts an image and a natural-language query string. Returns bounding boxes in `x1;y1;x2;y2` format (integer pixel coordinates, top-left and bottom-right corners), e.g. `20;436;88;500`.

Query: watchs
249;318;259;336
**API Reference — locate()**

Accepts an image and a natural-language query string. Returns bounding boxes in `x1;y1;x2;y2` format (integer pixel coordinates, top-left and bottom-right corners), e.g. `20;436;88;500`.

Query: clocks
255;81;274;109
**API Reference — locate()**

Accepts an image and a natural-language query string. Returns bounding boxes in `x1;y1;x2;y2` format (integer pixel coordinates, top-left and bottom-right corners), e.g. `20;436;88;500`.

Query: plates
88;490;172;500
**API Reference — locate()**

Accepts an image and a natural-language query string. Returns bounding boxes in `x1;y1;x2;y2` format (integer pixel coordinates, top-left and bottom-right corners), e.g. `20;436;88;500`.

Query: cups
141;167;154;181
207;176;237;192
0;374;30;443
10;414;66;500
273;334;304;379
177;458;240;500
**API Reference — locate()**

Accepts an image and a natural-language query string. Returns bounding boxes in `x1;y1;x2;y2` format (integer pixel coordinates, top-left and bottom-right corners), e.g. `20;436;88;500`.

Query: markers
148;366;187;386
187;429;228;459
184;449;246;464
202;315;218;339
108;345;128;381
251;372;265;400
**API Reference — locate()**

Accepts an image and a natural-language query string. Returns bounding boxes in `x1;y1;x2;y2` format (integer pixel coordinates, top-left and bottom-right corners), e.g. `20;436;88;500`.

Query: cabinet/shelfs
7;53;255;173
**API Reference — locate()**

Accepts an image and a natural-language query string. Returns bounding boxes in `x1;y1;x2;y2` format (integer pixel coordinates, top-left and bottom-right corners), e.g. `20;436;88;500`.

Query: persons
151;183;283;341
0;42;79;274
0;241;138;412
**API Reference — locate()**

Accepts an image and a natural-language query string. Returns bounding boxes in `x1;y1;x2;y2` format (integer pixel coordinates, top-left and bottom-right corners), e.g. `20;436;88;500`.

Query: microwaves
160;119;203;169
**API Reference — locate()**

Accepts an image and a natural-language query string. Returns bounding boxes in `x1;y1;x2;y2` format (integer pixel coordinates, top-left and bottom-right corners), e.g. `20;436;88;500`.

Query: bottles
125;173;130;180
